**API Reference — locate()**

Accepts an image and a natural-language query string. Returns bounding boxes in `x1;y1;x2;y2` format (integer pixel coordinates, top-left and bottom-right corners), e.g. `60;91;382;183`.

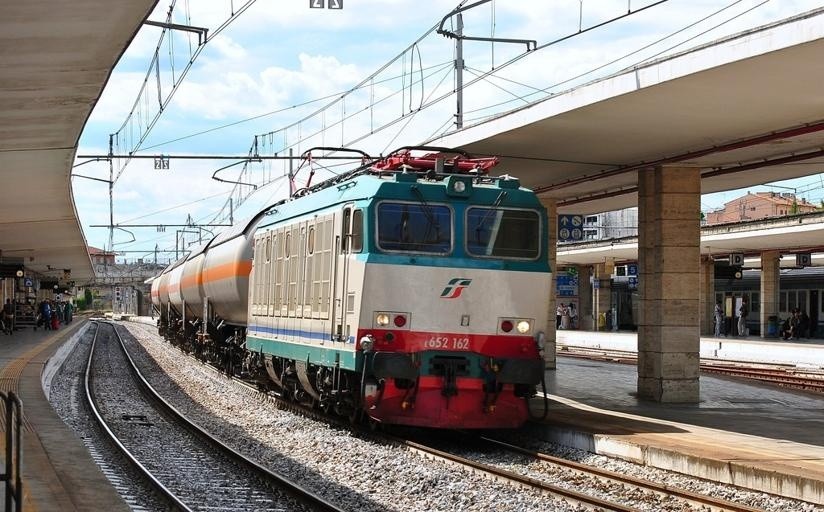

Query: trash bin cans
768;316;776;334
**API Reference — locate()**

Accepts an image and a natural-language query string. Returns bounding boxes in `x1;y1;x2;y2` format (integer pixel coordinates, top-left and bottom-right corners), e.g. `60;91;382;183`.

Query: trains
149;145;556;444
611;270;824;342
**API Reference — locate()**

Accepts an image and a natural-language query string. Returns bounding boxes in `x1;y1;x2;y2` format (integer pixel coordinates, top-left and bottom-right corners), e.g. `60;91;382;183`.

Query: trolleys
0;390;22;512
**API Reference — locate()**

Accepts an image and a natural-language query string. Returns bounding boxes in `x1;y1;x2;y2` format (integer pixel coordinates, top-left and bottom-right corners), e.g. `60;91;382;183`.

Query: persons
781;306;809;342
737;301;749;337
712;299;725;337
556;302;578;330
0;298;74;335
